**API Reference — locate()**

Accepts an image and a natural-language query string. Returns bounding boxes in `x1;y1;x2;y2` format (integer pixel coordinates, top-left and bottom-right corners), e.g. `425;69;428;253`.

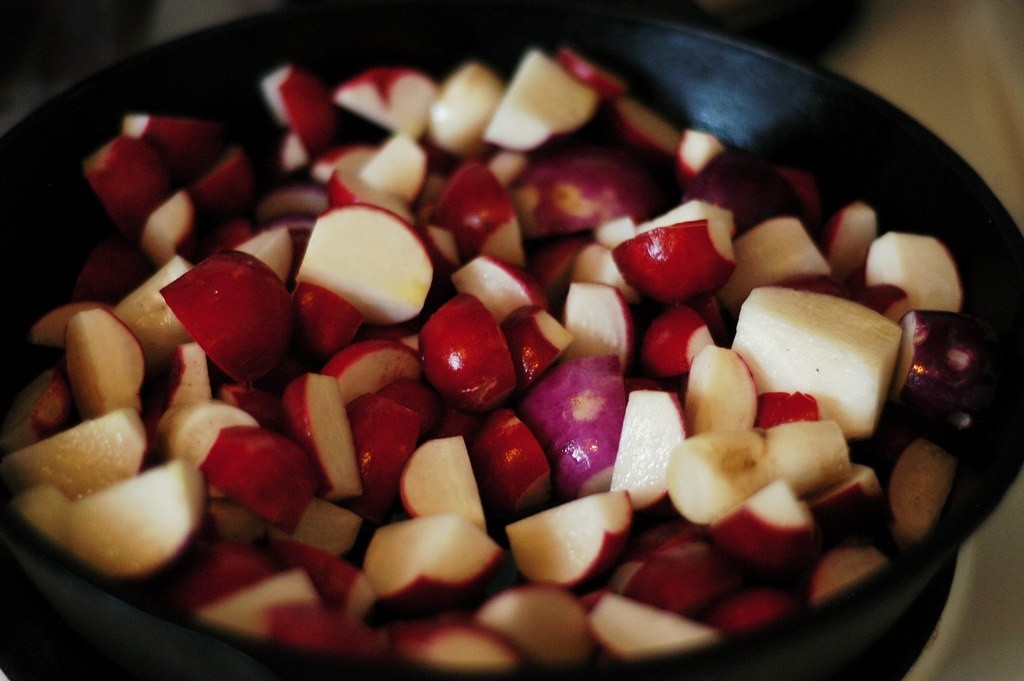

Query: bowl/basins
0;0;1024;681
